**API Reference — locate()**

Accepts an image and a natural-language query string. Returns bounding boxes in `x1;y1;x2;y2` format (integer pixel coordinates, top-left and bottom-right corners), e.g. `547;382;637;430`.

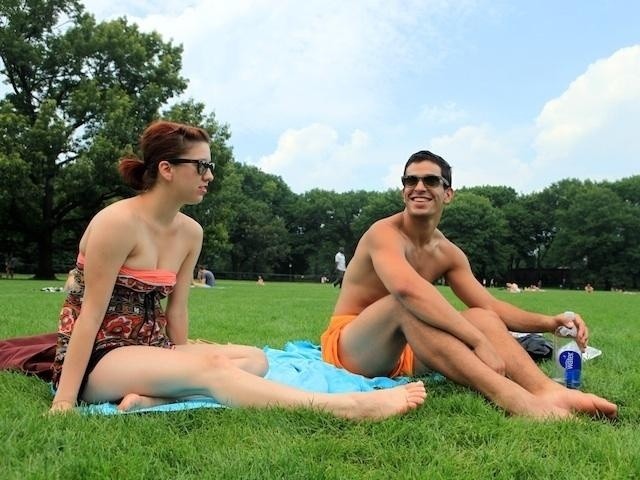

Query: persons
320;150;618;420
192;266;215;288
482;277;636;294
321;274;330;283
333;247;346;288
5;252;16;278
48;121;428;420
256;275;264;286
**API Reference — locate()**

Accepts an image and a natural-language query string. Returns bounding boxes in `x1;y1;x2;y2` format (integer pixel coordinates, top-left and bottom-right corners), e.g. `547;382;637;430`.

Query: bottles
555;312;588;389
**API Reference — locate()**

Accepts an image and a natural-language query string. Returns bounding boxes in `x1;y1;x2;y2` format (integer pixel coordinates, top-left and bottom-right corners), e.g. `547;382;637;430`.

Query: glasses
401;175;449;187
171;157;216;174
